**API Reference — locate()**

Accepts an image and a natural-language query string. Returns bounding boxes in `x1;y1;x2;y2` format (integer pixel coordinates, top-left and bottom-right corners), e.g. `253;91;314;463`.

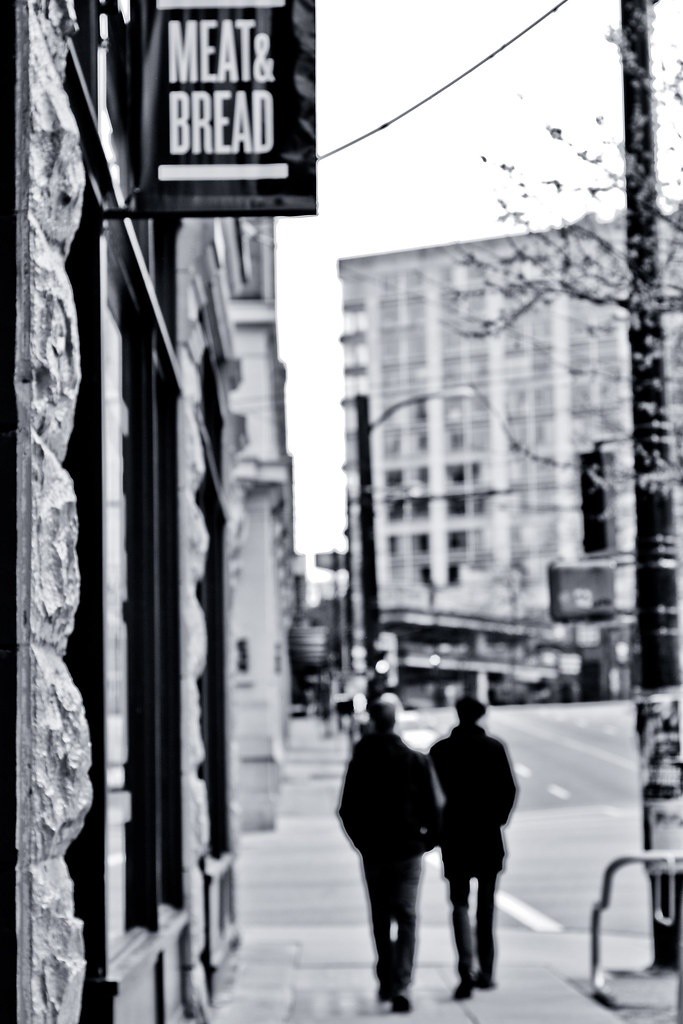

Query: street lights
352;383;474;705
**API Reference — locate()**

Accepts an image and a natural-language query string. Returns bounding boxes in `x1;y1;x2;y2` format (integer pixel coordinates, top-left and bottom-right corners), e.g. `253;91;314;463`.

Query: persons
336;694;446;1014
428;694;517;1000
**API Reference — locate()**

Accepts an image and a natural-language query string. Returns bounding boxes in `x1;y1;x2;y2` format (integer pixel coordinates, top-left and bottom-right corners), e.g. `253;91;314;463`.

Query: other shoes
392;994;410;1012
379;987;394;1001
476;970;493;988
455;976;475;999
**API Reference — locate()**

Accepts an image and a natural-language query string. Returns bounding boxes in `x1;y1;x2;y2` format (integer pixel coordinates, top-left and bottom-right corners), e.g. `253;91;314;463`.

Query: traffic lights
577;454;610;552
